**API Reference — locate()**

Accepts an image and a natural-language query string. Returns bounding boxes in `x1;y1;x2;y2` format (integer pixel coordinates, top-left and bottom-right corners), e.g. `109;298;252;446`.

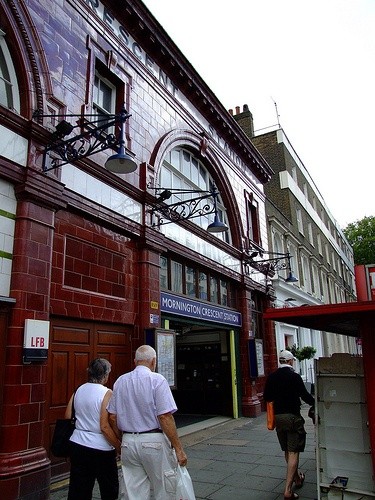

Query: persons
263;350;315;500
65;359;120;500
106;345;188;500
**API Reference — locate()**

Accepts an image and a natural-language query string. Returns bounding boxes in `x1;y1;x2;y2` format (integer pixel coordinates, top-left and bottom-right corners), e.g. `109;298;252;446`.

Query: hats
279;350;296;361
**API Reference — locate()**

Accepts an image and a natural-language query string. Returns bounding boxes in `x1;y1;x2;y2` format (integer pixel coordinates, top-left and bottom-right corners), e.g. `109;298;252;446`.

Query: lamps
144;188;229;233
249;252;259;258
32;112;140;175
156;191;172;203
245;251;298;283
52;119;74;139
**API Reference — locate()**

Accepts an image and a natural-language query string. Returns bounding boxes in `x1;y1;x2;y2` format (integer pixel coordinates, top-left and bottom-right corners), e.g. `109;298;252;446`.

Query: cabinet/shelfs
313;354;375;500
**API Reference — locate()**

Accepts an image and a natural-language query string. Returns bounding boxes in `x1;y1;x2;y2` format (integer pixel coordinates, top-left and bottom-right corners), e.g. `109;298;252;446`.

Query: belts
125;428;163;434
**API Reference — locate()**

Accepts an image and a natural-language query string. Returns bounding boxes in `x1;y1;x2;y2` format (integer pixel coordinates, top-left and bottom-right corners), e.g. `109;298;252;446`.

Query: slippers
284;492;298;500
296;473;305;488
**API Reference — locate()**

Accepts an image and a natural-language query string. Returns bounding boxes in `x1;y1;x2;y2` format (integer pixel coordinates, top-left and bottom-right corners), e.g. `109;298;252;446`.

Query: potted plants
287;345;318;374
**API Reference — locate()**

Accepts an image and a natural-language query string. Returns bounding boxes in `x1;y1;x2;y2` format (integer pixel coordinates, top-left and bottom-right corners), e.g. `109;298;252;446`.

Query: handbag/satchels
176;463;195;500
267;401;276;430
52;385;80;459
